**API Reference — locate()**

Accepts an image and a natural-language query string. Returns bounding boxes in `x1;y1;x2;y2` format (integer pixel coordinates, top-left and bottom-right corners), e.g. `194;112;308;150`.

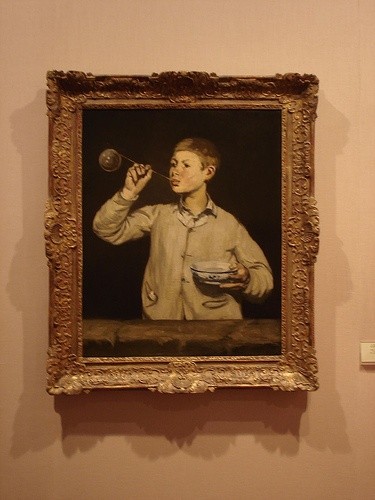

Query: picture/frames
44;66;324;395
